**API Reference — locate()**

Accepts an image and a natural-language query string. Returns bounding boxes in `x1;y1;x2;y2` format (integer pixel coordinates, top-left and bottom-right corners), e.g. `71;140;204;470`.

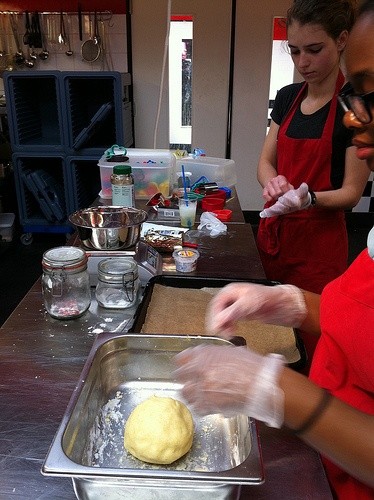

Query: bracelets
284;288;306;327
306;190;317;208
284;386;332;437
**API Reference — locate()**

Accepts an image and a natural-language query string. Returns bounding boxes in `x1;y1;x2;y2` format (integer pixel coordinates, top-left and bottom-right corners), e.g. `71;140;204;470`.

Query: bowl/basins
68;205;149;249
202;197;224;211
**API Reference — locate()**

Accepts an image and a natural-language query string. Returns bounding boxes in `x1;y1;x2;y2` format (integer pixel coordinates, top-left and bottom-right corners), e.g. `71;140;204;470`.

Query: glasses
338;81;374;124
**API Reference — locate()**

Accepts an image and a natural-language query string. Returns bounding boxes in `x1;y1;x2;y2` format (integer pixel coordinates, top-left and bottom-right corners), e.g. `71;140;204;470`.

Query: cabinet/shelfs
4;70;134;232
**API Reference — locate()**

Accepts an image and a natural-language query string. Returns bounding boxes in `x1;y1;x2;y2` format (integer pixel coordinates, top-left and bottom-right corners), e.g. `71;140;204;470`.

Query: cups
176;172;192;188
172;247;200;273
178;194;198;227
199;182;218;195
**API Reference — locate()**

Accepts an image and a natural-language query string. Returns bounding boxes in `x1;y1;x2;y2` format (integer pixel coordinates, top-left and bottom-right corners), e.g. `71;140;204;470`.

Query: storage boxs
97;149;174;199
176;154;237;188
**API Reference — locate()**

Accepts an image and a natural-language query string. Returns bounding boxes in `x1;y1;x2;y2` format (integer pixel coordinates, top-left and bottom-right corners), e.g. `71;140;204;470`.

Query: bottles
41;245;92;318
94;256;139;308
110;165;136;209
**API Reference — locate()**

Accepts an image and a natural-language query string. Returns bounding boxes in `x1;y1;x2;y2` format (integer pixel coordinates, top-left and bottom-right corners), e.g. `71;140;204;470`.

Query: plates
138;222;189;251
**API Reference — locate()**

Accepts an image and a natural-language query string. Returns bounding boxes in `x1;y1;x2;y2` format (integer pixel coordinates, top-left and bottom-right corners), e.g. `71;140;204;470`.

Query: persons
168;0;374;500
256;0;371;363
